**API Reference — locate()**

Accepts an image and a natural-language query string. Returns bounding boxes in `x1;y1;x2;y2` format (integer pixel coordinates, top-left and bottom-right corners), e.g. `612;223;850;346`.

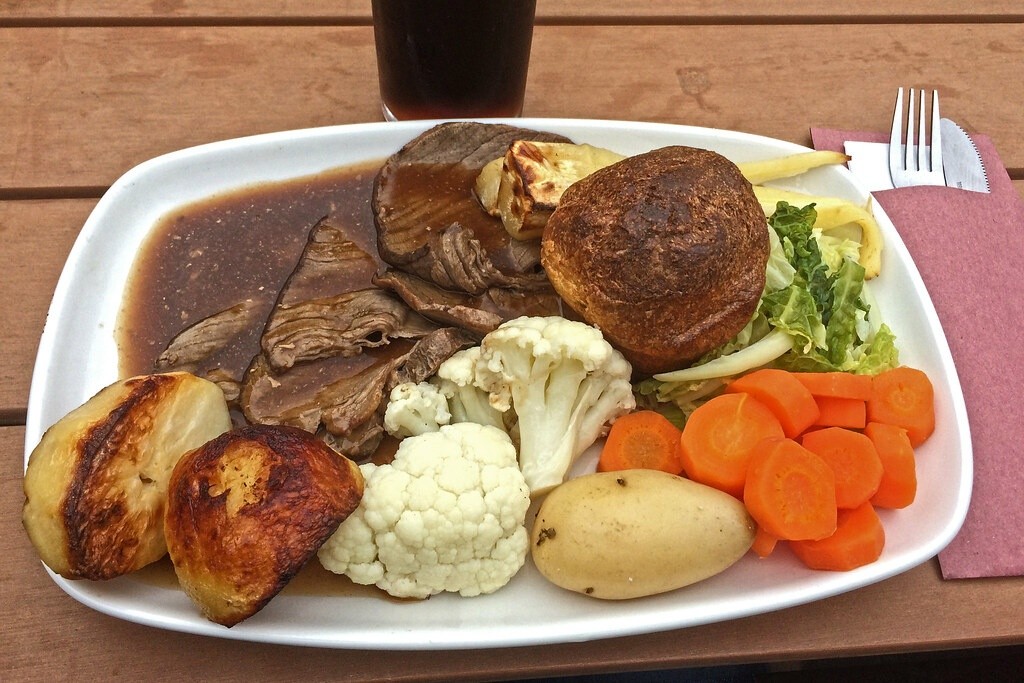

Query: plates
25;118;974;651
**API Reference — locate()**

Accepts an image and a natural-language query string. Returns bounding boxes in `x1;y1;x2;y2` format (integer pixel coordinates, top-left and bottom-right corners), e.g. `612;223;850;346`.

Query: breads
540;145;771;360
22;370;231;581
163;424;364;627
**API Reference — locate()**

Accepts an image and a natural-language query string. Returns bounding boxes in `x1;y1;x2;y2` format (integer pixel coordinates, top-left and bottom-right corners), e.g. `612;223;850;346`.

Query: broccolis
381;313;636;499
315;423;531;602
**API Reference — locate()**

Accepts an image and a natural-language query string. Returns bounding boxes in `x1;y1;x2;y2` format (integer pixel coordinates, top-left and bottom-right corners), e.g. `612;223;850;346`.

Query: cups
371;0;537;122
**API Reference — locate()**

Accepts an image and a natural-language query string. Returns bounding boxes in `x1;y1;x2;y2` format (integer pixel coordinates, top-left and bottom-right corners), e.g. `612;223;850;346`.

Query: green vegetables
631;201;897;431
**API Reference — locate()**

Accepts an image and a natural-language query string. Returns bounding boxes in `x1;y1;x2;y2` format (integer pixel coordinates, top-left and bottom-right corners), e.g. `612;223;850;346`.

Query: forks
890;87;944;188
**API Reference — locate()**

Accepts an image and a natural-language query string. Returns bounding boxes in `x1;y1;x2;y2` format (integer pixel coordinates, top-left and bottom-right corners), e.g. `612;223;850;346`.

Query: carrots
592;368;936;574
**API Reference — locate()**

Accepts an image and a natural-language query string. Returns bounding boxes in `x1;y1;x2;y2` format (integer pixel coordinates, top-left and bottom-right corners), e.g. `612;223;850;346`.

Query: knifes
940;117;991;193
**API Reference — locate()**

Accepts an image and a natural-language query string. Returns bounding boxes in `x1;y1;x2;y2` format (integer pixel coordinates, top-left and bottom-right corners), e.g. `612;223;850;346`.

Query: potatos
531;469;757;600
